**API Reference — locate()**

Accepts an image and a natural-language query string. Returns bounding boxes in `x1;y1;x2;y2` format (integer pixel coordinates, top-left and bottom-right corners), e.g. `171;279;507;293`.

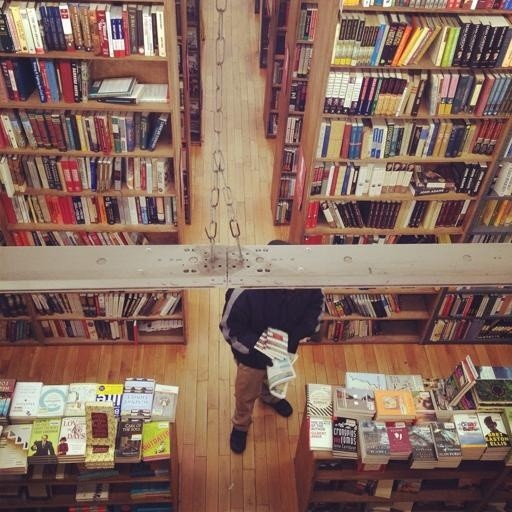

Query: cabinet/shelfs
0;419;182;512
293;384;512;511
252;0;511;345
1;0;207;347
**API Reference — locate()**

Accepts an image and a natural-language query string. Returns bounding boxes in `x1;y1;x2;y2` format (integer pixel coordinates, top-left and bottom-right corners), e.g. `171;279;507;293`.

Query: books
306;355;511;511
323;294;400;342
259;1;318;227
430;292;511;342
265;326;289;359
2;376;179;510
457;286;505;291
262;336;285;361
306;355;512;511
254;329;275;361
4;0;200;245
343;1;510;9
4;291;181;342
304;11;511;246
305;235;510;245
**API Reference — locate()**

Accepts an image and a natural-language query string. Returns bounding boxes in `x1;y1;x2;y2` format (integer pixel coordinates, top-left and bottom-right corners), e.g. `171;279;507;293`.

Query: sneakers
228;426;247;455
270;398;293;418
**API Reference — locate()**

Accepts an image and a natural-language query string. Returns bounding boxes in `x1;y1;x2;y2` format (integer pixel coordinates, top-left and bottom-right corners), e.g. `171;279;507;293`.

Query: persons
401;163;407;171
57;437;69;455
386;163;393;171
394;163;401;171
30;435;55;455
407;163;414;171
422;165;448;179
219;240;324;454
